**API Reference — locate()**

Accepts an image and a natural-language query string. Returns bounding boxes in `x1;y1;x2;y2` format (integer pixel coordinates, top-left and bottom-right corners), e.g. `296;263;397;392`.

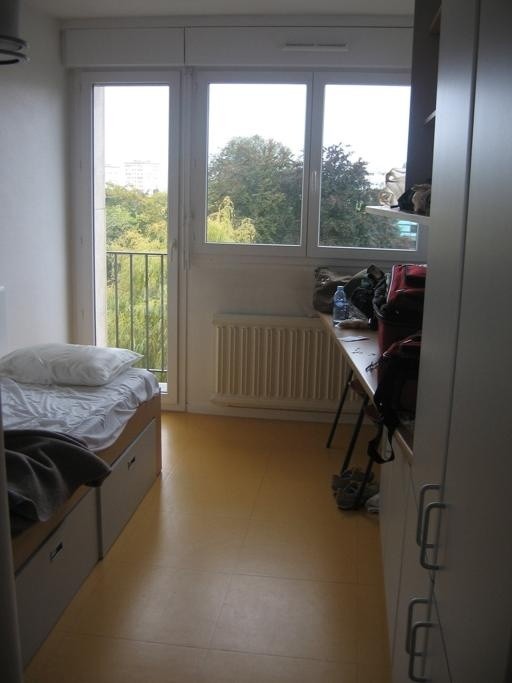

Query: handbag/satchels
313;265;386;314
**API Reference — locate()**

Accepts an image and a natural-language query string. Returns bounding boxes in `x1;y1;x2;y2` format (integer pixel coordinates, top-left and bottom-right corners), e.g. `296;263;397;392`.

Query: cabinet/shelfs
15;417;159;669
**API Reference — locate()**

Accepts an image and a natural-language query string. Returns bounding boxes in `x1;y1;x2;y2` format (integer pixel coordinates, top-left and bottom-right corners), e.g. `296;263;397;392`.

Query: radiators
205;311;372;414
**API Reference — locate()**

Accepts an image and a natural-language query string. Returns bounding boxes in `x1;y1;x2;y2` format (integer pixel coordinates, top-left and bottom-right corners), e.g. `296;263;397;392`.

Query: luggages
372;264;427;354
378;330;422;412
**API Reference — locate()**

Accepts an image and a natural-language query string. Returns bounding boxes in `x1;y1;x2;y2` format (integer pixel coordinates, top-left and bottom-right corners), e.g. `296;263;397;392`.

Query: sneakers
336;481;378;510
333;469;374;490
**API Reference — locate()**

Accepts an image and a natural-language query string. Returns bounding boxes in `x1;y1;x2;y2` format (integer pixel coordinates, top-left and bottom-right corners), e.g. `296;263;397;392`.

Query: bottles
333;285;348;325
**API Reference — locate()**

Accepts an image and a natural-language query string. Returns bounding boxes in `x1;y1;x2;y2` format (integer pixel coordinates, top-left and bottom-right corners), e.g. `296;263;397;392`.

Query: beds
0;366;163;669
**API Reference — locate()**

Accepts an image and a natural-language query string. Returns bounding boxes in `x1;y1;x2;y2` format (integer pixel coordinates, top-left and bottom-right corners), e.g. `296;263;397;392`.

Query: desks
313;311;412;498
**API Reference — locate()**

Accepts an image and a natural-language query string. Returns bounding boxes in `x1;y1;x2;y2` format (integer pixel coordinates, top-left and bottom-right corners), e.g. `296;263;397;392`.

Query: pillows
2;340;145;388
